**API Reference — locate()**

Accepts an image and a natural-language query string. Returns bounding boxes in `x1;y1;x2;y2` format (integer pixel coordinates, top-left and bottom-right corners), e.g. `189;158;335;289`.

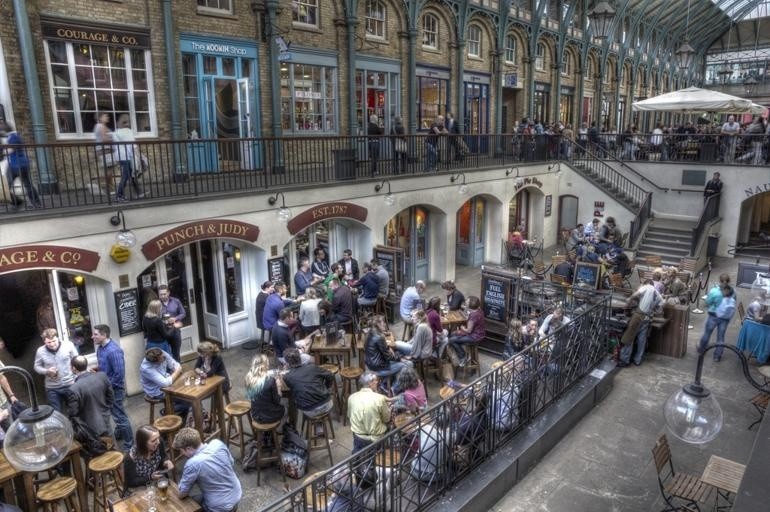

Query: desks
735;317;770;367
699;454;747;512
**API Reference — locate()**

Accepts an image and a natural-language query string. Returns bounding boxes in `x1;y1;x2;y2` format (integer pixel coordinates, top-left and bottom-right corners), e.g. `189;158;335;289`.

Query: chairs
259;281;529;512
650;433;713;512
503;227;701;300
738;302;745;322
1;364;285;511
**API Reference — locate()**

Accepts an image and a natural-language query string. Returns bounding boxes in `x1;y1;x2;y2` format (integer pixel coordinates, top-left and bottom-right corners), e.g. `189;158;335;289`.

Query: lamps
269;190;292;223
585;0;764;92
374;178;397;206
450;172;470;195
547;161;564;179
505;165;523;186
111;208;136;249
661;342;770;445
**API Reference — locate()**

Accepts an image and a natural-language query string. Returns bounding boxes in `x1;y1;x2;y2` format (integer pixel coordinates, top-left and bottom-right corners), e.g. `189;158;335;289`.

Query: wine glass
315;326;326;347
163;314;173;328
440;304;450;320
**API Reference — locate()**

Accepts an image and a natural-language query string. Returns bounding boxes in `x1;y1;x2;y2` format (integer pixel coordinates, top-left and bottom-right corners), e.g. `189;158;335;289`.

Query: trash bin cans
332;148;356;181
700;142;718;163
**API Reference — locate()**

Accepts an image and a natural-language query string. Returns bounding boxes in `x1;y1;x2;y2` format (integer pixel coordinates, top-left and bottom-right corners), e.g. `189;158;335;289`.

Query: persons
366;114;459;176
512;114;770;165
93;112;120;195
0;123;45;212
112;114;151;202
703;172;723;206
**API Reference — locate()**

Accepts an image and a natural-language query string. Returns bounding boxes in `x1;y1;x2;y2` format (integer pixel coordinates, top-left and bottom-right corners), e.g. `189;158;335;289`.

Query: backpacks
715;295;736;321
278;422;310;479
241;439;259;473
184;404;211;433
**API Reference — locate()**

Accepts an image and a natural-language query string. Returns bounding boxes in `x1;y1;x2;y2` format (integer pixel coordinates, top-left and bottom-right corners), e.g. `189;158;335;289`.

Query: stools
746;390;769;431
758;365;770;388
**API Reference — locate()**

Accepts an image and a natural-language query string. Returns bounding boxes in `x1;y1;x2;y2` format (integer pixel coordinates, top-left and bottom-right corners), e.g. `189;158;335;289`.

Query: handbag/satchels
435;359;456;383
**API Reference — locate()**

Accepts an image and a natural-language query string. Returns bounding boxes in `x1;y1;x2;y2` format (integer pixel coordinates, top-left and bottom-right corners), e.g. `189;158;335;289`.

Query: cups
146;480;169;512
273;368;287;386
184;372;207;387
91;361;99;373
50;364;57;379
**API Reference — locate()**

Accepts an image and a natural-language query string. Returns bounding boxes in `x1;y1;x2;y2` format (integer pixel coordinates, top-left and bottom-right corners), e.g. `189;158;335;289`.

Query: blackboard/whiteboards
480;273;511;327
114;287;143;337
573;261;601;290
268;256;285;282
373;247;397;289
